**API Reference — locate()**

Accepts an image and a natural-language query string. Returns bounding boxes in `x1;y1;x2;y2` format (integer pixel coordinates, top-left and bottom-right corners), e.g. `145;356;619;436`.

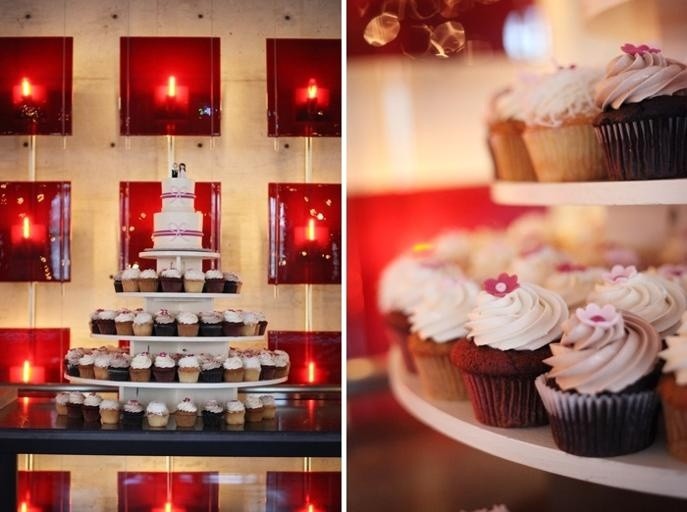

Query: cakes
109;264;243;294
53;392;277;430
483;40;687;183
62;344;289;384
87;307;269;338
377;217;687;461
153;179;202;249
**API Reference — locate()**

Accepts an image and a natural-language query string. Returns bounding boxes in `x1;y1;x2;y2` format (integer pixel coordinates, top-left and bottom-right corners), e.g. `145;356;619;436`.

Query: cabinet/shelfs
61;248;290;387
371;177;685;502
